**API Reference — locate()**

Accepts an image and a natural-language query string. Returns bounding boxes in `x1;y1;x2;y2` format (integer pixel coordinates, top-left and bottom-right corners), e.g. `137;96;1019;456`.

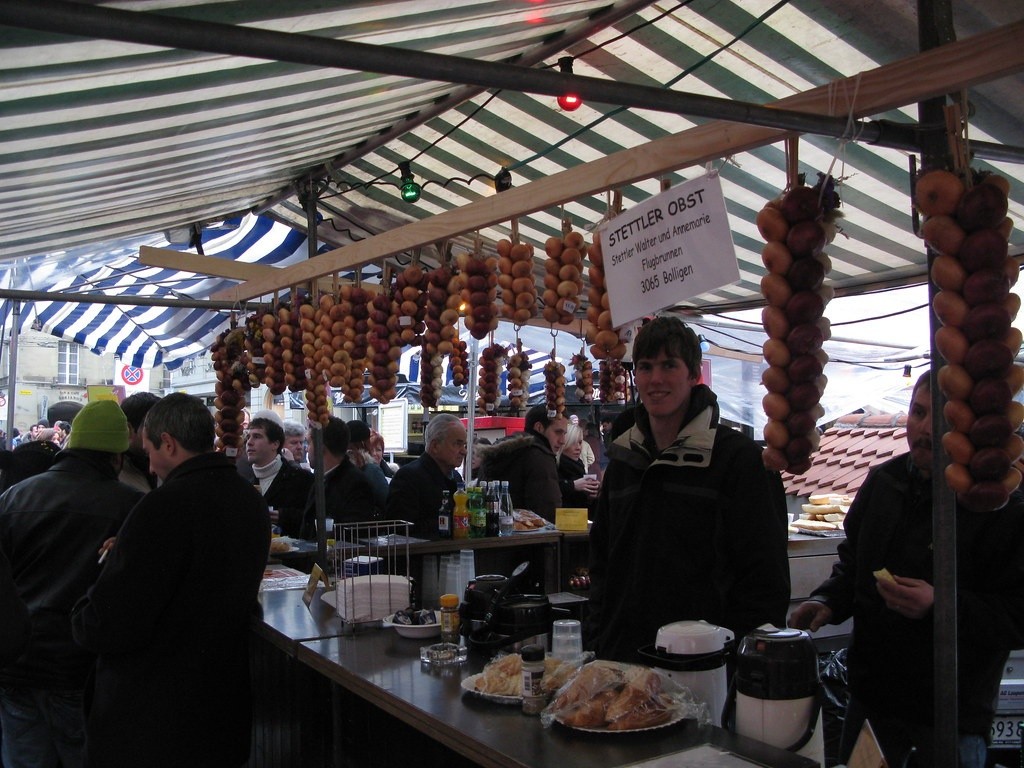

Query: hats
347;421;369;441
37;419;48;429
70;399;129;453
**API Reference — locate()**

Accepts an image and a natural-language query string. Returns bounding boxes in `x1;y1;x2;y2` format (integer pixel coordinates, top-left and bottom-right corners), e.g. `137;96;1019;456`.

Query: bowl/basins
382;610;442;638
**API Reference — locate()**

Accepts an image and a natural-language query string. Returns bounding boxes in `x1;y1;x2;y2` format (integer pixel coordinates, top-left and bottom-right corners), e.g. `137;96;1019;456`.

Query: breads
873;567;898;583
554;663;674;731
512;509;546;530
271;539;289;551
787;494;854;533
475;654;574;696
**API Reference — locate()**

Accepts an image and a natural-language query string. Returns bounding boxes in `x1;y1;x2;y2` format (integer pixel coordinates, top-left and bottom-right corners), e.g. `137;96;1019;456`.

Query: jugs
719;622;825;768
637;620;736;725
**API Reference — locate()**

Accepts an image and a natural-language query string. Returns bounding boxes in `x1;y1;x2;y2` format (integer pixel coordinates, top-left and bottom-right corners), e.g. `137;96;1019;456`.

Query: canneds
439;593;460;644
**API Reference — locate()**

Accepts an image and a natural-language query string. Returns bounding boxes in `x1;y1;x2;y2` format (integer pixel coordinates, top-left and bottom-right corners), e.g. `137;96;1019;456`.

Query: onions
915;169;1024;512
212;232;632;455
756;187;836;476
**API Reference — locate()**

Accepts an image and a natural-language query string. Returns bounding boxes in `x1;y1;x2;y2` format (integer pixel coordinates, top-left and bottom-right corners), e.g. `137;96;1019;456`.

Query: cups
315;518;333;549
552;619;583;673
423;549;475;609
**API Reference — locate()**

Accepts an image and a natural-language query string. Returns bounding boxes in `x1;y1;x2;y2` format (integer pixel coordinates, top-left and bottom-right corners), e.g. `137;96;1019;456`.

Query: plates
553;711;686;732
277;546;300;553
461;674;523;706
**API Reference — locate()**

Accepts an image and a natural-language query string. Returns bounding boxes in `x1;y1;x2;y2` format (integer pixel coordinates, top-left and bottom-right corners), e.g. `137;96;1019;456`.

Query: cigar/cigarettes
98;543;111;563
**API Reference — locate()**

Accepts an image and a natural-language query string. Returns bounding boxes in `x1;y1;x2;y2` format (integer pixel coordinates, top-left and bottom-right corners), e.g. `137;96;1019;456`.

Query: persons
0;390;396;768
377;413;467;608
582;318;791;663
468;401;615;524
787;371;1024;768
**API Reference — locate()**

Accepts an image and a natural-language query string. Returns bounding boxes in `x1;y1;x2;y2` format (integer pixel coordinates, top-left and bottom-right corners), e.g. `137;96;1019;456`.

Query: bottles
521;644;547;716
439;593;460;645
271;524;281;538
439;478;513;540
